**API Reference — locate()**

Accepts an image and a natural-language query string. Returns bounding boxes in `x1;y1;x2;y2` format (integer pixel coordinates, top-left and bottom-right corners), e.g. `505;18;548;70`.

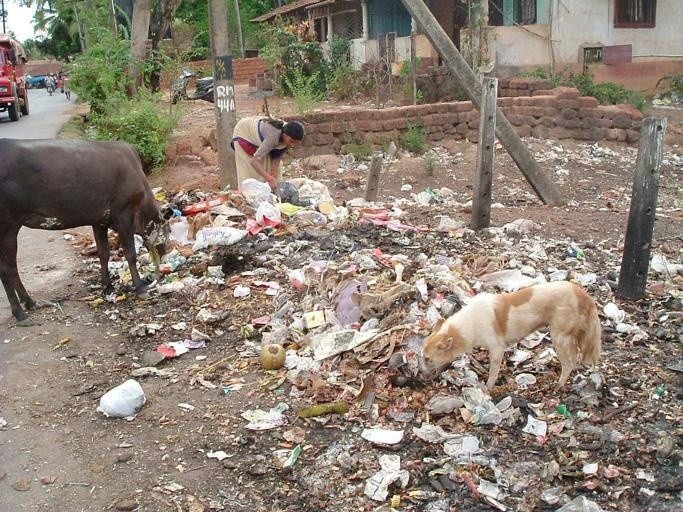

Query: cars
25;76;44;88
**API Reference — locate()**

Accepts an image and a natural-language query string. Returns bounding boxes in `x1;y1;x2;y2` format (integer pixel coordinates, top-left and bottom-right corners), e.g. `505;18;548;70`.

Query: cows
0;138;166;327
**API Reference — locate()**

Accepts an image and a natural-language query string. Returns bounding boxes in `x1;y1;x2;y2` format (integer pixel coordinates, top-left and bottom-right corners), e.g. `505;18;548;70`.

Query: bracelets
268;176;273;182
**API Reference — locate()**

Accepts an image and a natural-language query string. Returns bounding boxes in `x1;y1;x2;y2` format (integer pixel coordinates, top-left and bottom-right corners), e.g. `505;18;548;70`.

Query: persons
45;70;70;102
231;115;305;191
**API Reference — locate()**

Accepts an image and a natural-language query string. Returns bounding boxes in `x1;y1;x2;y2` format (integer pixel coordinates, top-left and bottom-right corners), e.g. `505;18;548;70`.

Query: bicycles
46;80;54;96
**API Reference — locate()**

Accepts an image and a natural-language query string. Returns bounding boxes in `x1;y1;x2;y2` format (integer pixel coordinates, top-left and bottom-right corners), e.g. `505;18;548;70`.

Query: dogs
417;280;603;395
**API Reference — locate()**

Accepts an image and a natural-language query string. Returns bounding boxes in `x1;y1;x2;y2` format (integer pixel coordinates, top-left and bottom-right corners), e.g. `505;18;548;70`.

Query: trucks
0;33;28;120
